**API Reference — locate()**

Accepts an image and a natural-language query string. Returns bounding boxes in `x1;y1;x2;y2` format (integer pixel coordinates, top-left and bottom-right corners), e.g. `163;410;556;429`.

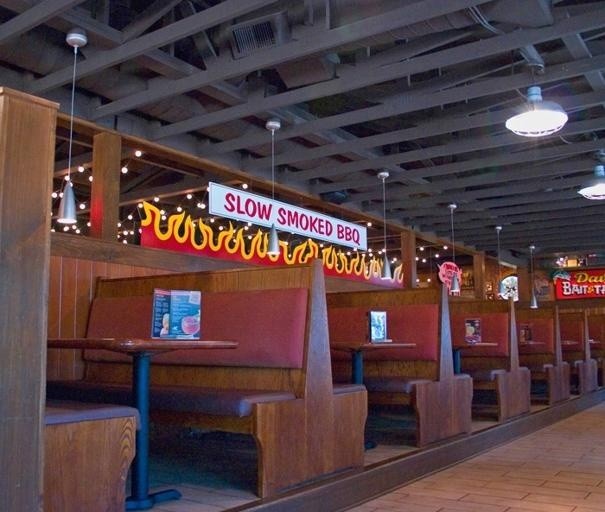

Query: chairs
325;283;474;447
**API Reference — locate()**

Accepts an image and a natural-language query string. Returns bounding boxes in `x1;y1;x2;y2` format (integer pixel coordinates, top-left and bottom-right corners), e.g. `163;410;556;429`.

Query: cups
181;317;200;336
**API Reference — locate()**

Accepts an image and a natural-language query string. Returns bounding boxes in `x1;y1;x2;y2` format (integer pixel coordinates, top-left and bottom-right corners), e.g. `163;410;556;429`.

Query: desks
331;341;416;451
43;335;240;510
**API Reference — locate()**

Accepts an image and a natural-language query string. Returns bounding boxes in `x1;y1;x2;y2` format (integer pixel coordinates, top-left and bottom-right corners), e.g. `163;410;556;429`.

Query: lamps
577;151;605;202
377;172;392;281
504;63;570;138
447;204;539;310
265;117;281;255
55;29;89;227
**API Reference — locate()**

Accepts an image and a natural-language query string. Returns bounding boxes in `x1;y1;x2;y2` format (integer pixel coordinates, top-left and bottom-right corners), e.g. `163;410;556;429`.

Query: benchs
45;260;368;500
453;258;604;512
46;399;143;511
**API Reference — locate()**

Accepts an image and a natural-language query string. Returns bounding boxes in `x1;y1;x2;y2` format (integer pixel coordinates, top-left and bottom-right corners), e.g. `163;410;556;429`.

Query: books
464;319;481;343
368;311;387;343
150;288;202;340
519;324;530;342
